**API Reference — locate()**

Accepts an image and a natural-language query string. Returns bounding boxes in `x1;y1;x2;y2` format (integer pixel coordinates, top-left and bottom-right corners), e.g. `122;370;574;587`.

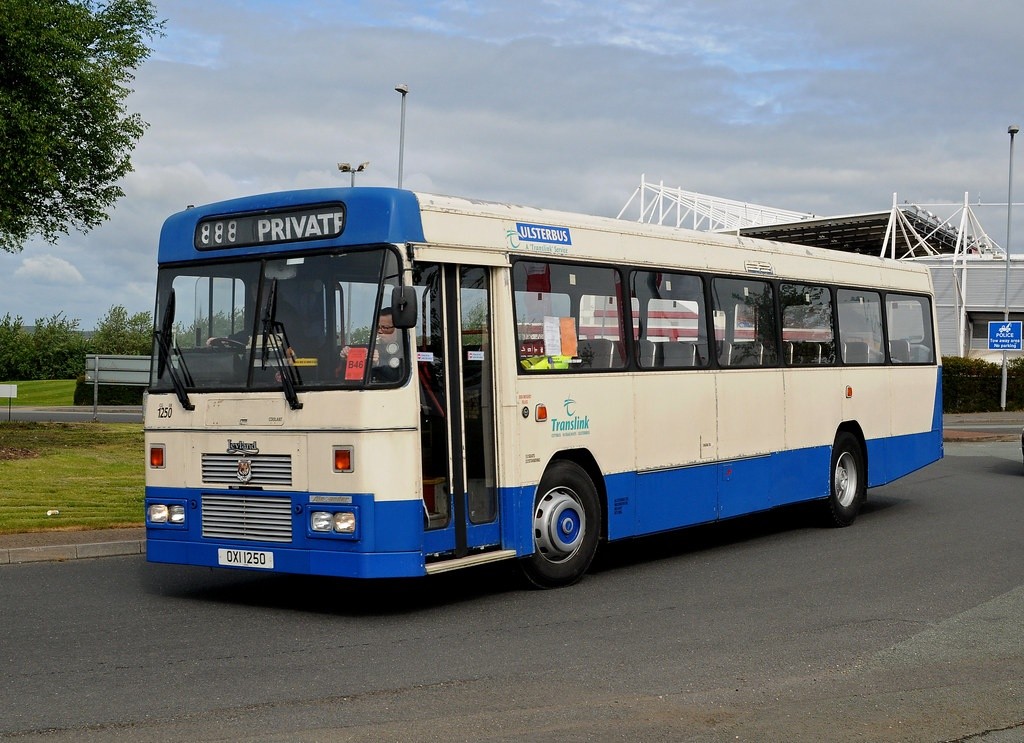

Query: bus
144;188;943;590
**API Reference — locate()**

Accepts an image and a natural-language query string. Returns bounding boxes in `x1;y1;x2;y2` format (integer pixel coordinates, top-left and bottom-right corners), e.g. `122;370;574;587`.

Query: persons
206;271;309;358
340;307;403;383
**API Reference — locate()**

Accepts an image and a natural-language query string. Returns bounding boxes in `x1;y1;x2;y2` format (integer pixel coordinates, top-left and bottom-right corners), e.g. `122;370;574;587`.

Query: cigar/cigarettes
376;337;381;340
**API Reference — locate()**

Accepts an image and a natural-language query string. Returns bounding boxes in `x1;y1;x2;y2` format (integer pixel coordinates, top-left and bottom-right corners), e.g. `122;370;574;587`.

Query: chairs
568;340;911;369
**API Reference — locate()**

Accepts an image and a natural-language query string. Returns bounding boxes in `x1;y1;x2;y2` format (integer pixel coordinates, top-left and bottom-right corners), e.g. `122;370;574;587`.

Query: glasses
377;326;394;331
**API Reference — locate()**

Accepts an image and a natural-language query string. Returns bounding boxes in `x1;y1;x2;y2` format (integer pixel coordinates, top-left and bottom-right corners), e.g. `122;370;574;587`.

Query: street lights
1000;125;1019;411
338;161;369;187
394;83;409;189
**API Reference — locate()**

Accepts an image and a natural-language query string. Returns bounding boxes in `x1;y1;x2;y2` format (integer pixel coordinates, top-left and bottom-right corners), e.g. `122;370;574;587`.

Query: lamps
912;204;987;247
